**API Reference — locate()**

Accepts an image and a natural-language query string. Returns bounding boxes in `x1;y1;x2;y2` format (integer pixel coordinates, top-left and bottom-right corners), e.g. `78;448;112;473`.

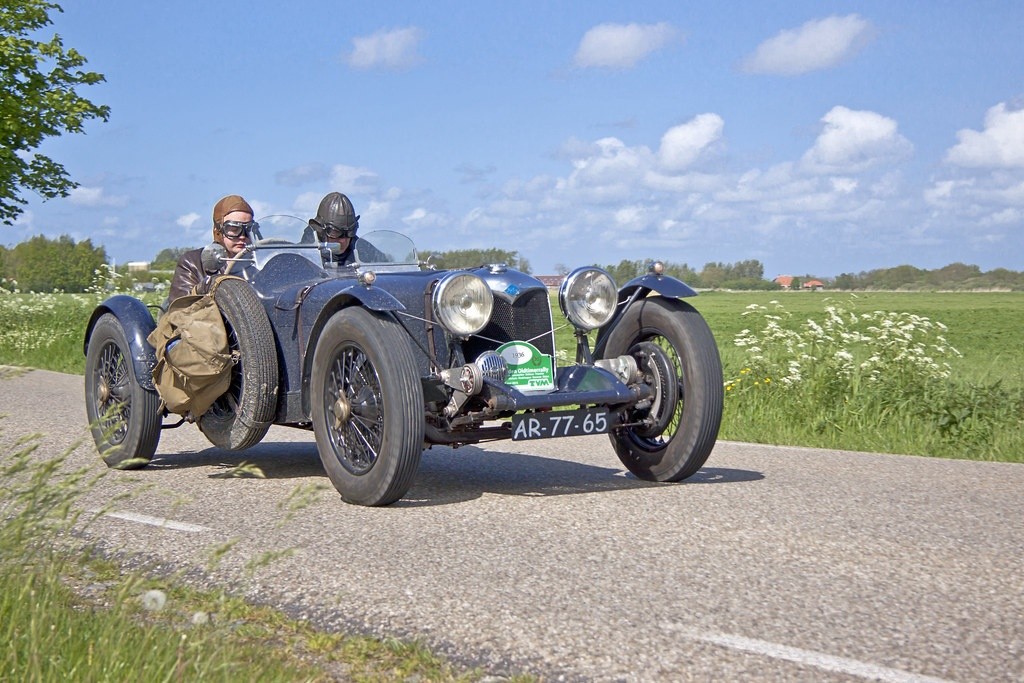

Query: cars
82;214;725;506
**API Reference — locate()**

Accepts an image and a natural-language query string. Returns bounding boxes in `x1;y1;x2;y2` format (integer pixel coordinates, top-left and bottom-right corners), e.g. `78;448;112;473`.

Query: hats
318;192;356;230
213;195;253;243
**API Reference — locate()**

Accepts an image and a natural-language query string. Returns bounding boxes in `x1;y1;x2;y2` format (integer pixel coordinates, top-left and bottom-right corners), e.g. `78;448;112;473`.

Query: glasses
325;223;359;238
222;221;255;238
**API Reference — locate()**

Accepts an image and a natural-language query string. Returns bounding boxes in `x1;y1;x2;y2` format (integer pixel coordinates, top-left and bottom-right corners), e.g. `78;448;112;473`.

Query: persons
168;194;259;306
295;191;393;267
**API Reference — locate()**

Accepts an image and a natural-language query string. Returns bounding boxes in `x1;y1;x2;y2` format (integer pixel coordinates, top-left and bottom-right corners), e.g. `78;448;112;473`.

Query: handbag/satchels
145;294;235;425
